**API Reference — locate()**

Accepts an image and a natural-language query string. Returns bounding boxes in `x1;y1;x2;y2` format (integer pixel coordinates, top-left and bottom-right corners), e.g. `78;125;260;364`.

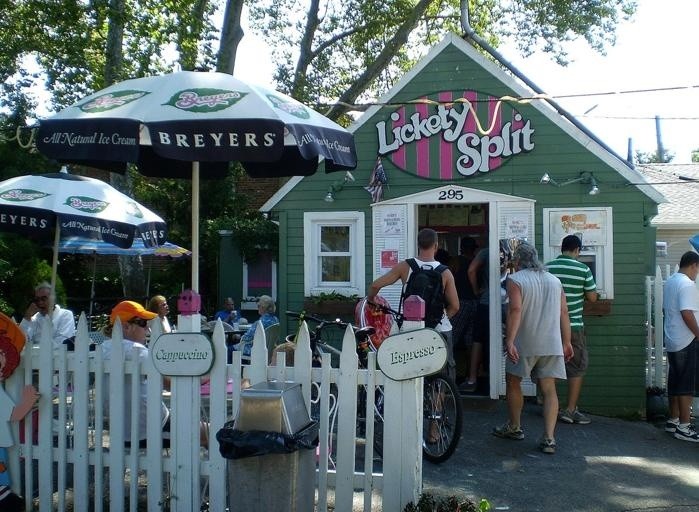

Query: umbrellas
36;70;357;292
0;170;166;318
54;234;191;335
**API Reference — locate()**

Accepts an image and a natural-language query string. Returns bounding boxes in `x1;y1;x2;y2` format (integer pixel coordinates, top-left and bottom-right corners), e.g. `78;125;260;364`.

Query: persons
145;295;174;337
94;300;210;451
491;242;574;455
457;238;491;393
17;283;76;352
543;235;600;426
662;250;699;443
212;297;246;345
366;228;461;446
226;294;280;365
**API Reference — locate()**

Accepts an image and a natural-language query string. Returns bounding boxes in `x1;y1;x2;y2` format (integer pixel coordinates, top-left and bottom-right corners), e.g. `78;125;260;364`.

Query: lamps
539;172;584;188
588;177;601;195
323;184;335;203
339;171;355;187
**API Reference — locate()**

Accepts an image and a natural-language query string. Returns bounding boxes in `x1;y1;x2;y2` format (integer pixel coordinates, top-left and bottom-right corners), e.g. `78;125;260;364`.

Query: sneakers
493;422;525;442
536;391;544;405
673;424;699;443
466;378;478;388
538;436;557;454
560;406;593;425
665;420;680;434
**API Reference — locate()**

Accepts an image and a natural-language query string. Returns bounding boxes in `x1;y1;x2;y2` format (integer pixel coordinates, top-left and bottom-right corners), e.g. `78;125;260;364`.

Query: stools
124;439;170;508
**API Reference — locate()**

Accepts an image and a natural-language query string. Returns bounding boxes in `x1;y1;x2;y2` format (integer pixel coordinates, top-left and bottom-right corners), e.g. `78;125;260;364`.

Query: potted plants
303;289;363;314
239;295;260;310
646;386;666;415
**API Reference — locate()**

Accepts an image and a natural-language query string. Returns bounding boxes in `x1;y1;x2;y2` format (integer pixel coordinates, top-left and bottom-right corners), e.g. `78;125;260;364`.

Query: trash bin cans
216;381;319;511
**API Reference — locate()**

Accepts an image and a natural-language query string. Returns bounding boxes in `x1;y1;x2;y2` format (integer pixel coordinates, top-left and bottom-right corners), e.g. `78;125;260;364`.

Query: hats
111;300;159;327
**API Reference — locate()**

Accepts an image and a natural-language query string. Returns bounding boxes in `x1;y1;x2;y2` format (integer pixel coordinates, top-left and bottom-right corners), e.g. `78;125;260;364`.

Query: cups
234;323;239;331
231;311;237;319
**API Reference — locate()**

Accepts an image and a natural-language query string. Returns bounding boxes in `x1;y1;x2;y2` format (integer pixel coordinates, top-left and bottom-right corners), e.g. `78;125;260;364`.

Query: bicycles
283;311;384;456
366;301;463;462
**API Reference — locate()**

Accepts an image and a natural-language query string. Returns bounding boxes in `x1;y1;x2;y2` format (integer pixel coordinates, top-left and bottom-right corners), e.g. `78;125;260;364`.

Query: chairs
239;322;281;367
53;336;97;450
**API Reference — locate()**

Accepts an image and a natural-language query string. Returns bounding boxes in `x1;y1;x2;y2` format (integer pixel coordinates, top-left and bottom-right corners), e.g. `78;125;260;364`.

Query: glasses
257;304;261;308
32;295;50;303
128;318;148;328
228;304;234;306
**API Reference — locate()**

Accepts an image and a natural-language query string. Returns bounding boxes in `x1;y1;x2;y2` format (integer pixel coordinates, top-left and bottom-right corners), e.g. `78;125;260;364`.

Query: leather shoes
427;430;448;446
